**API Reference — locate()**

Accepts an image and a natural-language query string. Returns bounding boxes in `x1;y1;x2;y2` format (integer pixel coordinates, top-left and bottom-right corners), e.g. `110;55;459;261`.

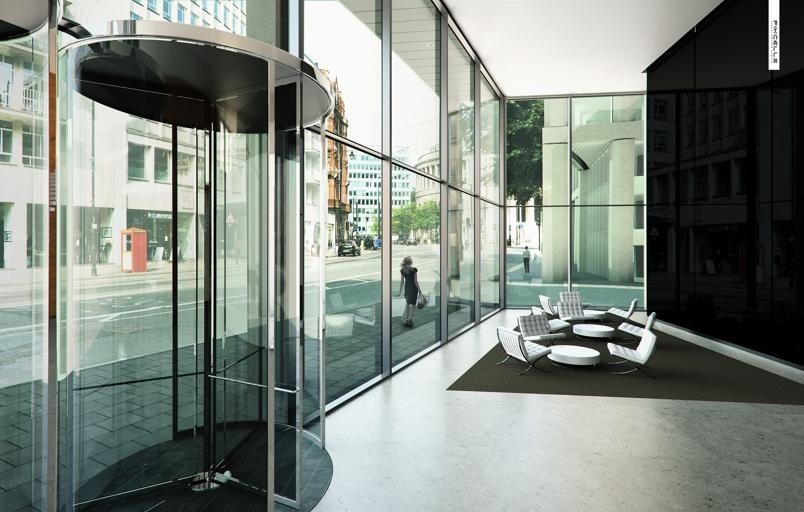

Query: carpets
447;314;803;405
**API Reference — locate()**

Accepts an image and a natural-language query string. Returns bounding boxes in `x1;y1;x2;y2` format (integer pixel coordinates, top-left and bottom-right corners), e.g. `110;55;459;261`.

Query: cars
338;233;440;260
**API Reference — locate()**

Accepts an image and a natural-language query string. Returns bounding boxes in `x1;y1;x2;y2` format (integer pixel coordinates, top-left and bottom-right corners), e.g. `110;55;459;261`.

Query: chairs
324;294;436;337
497;327;553;376
607;330;657;375
519;292;657;347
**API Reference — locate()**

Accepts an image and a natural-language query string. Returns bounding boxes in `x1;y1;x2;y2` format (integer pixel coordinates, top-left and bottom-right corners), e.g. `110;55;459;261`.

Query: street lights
336;148;355;243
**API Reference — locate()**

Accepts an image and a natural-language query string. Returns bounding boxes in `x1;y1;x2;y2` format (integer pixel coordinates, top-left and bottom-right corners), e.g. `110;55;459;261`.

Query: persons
521;246;530;274
396;256;422;327
